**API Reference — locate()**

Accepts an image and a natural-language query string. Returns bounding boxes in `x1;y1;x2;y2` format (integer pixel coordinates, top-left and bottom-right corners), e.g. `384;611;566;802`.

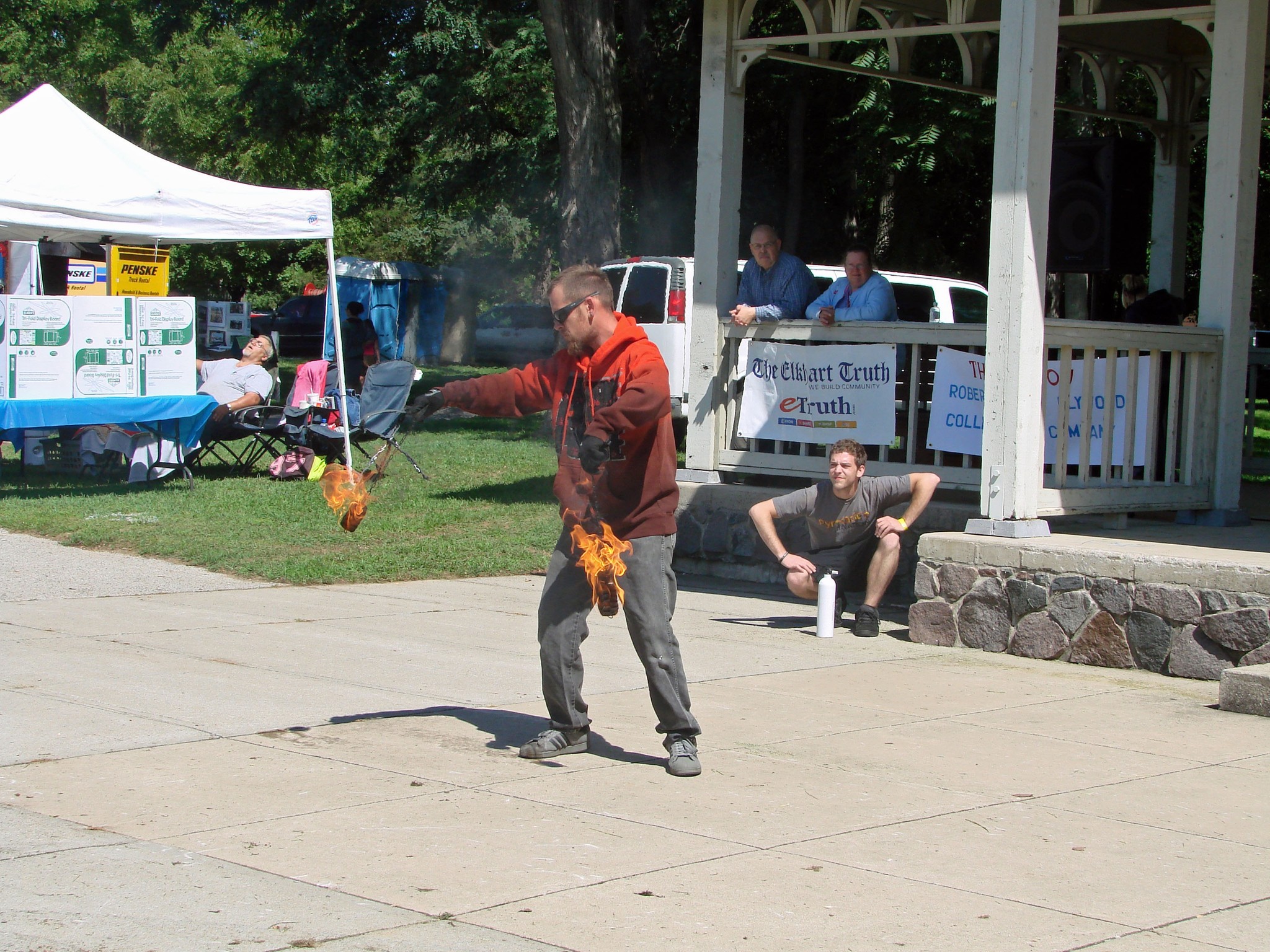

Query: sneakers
666;735;702;777
855;604;880;637
834;591;848;627
518;723;592;759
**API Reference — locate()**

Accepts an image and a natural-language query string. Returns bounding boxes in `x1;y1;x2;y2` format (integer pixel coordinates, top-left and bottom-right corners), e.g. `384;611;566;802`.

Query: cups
307;393;319;404
299;400;308;409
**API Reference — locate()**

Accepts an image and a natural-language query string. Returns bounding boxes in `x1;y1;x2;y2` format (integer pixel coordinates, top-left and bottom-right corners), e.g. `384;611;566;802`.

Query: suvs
250;295;327;357
474;303;558;349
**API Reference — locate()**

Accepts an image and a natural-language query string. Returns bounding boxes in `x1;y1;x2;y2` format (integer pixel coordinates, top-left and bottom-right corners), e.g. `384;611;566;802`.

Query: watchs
226;403;232;414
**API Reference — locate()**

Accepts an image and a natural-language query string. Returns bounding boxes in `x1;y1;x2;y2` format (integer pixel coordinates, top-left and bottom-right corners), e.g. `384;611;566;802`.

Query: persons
177;335;273;475
728;224;904;458
409;266;705;776
748;439;941;638
359;319;381;391
1109;274;1198;480
335;301;367;394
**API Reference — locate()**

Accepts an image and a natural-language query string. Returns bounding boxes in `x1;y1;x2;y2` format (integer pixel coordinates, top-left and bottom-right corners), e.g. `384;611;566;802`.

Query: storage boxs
0;292;253;400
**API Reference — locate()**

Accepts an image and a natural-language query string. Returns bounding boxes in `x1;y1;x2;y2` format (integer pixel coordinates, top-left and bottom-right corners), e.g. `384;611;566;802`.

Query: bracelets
779;552;788;563
818;309;822;319
897;518;909;531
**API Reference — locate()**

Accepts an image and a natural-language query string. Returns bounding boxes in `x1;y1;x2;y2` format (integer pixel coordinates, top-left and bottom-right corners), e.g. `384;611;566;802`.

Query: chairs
228;362;339;479
304;359;430;480
184;363;280;475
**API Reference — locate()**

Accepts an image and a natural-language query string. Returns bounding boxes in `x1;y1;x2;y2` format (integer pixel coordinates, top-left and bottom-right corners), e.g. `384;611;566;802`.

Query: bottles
816;566;838;638
320;418;329;428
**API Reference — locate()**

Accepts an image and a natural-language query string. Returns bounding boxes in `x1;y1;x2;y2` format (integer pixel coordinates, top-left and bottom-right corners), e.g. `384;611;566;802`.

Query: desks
0;395;219;492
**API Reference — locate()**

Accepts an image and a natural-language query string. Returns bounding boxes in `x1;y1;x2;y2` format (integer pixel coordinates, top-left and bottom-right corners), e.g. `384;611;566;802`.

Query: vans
598;256;987;418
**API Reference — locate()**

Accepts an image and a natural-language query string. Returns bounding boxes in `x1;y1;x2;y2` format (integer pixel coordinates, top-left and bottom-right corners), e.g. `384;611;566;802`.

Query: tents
0;82;446;486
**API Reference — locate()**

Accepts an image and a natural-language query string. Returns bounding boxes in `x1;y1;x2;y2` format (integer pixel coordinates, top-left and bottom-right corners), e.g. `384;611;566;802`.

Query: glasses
553;290;600;323
249;338;268;355
750;240;778;249
